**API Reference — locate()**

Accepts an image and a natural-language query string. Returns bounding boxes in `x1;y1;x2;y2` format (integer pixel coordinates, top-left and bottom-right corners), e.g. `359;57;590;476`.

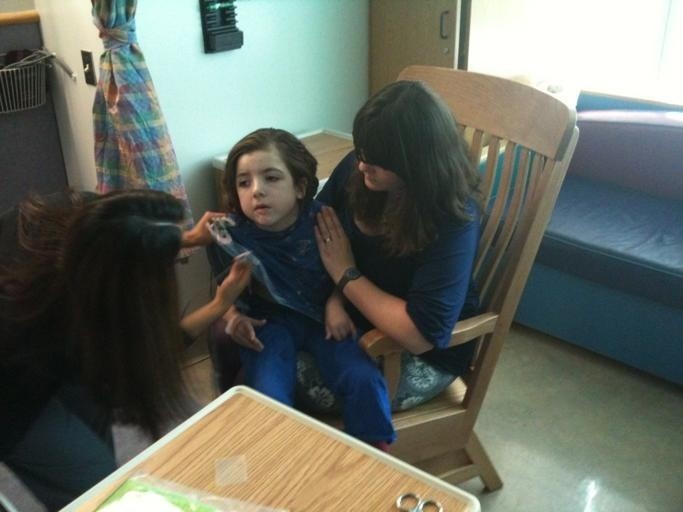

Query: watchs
334;266;362;292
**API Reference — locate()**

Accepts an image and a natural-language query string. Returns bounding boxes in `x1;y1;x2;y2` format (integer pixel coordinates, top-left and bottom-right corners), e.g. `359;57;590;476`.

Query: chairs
182;62;584;492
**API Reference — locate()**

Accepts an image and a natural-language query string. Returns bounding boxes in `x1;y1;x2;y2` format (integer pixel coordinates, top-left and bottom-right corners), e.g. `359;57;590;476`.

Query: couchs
474;89;682;388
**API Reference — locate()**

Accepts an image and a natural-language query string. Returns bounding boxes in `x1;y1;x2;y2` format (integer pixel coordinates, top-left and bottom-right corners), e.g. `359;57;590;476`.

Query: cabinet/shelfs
366;0;474;99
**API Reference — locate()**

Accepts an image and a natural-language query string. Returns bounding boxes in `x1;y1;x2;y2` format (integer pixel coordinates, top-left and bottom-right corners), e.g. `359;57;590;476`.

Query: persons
288;77;483;423
0;188;256;505
217;128;399;463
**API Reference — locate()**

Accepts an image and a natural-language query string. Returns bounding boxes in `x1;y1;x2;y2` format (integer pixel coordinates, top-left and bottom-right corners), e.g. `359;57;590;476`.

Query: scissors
397;493;444;512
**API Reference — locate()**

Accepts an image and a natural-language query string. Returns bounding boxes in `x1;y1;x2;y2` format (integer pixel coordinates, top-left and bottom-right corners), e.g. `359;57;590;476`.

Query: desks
53;382;484;512
213;126;358;216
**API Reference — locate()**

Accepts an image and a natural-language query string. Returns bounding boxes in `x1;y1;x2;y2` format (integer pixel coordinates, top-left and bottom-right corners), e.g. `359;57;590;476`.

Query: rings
323;237;332;243
